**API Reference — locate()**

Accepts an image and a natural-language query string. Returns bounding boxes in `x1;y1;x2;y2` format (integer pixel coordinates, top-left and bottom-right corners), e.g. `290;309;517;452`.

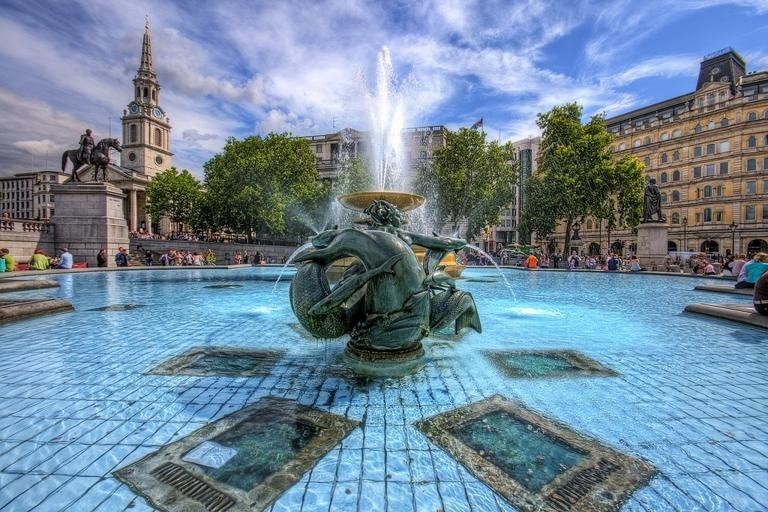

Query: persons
51;247;73;269
744;252;768;288
642;178;666;223
566;250;581;268
630;255;642;272
0;249;6;273
114;247;126;267
129;229;269;266
751;270;768;316
27;247;49;270
567;259;576;272
526;253;539;268
1;248;16;272
691;250;758;278
539;250;566;269
735;253;757;288
79;129;96;166
4;210;9;225
97;248;108;267
581;252;623;271
118;249;130;266
460;250;490;266
268;255;288;264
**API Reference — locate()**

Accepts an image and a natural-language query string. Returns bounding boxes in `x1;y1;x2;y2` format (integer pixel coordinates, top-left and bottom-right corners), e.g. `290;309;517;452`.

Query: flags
471;119;484;131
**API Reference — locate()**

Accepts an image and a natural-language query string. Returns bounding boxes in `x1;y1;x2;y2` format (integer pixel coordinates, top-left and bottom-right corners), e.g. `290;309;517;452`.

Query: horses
61;137;123;182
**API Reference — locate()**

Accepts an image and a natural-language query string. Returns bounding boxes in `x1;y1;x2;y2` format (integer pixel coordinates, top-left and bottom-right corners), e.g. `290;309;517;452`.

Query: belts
753;299;768;305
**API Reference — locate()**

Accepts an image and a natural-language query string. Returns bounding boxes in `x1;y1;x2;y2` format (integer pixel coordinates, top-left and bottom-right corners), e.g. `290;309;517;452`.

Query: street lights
682;216;687;252
728;221;738;259
